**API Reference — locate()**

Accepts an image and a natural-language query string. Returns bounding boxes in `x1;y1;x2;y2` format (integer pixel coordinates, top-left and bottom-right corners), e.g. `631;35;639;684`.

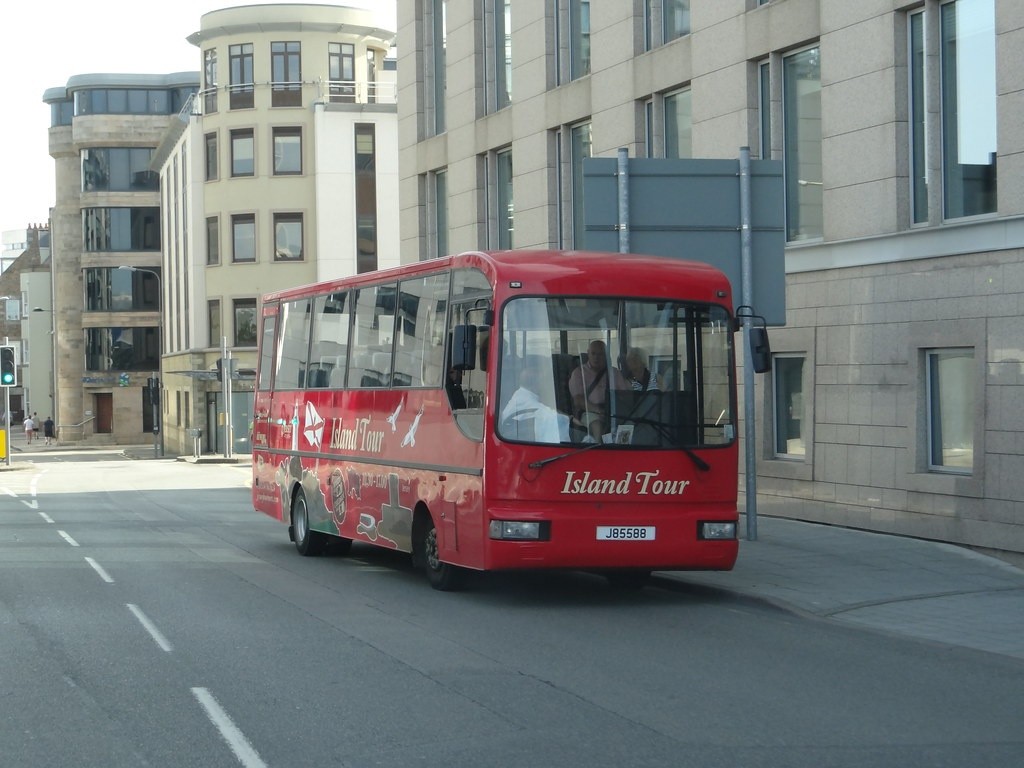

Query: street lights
118;266;166;457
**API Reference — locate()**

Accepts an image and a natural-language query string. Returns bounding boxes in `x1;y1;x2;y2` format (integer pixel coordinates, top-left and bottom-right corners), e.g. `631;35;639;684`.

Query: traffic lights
1;346;17;388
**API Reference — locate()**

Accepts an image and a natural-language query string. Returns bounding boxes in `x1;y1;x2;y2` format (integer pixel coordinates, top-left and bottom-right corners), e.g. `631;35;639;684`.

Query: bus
252;250;771;592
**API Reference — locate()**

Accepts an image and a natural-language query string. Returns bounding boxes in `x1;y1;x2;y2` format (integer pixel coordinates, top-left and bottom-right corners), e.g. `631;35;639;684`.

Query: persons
499;368;558;441
43;416;55;445
31;412;40;440
565;339;634;443
24;415;34;444
625;348;659;390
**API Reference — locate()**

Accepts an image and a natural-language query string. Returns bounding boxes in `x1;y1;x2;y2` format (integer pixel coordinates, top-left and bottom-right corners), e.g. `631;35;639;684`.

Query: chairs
317;340;576;397
